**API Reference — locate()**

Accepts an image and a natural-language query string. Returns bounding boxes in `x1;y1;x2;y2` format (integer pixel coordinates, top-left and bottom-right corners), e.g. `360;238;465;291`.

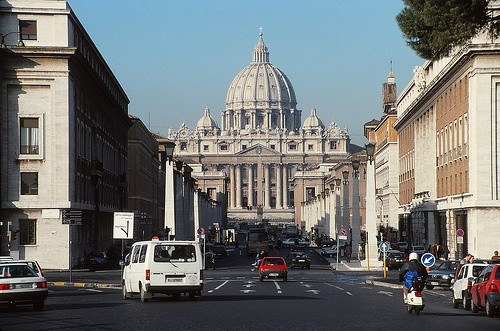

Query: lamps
336;178;341;191
365;142;376;165
342;170;350;185
159;141;176;165
351;160;361;177
301;182;334;207
176;160;184;178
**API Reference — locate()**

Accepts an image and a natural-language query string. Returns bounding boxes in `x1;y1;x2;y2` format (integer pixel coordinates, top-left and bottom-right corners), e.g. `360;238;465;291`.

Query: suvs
449;262;490;311
470;263;500;317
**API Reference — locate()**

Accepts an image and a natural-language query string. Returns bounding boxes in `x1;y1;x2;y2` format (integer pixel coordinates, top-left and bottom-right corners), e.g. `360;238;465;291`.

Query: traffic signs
62;209;82;224
113;212;134;239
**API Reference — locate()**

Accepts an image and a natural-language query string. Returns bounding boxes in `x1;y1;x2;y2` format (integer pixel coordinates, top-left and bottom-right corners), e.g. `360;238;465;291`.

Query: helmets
409;252;418;261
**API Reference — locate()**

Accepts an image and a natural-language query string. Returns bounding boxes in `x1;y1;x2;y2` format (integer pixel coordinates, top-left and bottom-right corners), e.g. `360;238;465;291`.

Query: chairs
161;250;169;259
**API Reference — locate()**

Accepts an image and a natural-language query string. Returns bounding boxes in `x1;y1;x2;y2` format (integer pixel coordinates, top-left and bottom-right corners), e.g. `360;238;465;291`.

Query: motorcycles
401;275;427;316
249;253;261;272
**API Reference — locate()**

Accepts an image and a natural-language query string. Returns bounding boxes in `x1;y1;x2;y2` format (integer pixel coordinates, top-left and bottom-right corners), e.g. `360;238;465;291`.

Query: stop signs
152;236;160;241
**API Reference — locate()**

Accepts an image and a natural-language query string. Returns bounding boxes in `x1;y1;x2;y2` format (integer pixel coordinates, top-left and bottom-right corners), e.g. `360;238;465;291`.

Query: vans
119;240;204;303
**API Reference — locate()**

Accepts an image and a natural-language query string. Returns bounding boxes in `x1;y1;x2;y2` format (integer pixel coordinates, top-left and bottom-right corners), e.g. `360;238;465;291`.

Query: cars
0;256;43;277
377;241;426;270
257;256;288;282
0;263;48;310
88;241;137;268
425;259;461;291
201;239;241;270
278;223;340;270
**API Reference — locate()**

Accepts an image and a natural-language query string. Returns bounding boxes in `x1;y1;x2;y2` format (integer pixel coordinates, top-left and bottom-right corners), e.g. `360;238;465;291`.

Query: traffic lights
11;230;17;240
383;233;388;242
376;235;381;247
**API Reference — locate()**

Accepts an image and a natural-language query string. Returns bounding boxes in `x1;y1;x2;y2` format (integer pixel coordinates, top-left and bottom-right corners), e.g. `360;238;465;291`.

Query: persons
162;250;195;261
464;254;474;260
257;250;266;265
492;251;500;260
277;238;282;249
340;242;362;263
447;258;460;265
399;252;427;299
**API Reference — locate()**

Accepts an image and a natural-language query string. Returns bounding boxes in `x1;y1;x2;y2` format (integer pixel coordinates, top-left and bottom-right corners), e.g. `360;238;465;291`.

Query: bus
245;228;269;255
